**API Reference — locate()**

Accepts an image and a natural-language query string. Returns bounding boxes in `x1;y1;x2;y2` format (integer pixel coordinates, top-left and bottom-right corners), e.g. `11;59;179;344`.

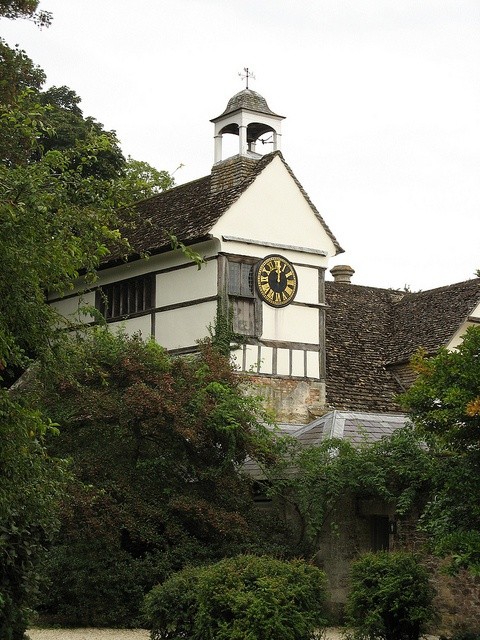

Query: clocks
255;255;298;309
